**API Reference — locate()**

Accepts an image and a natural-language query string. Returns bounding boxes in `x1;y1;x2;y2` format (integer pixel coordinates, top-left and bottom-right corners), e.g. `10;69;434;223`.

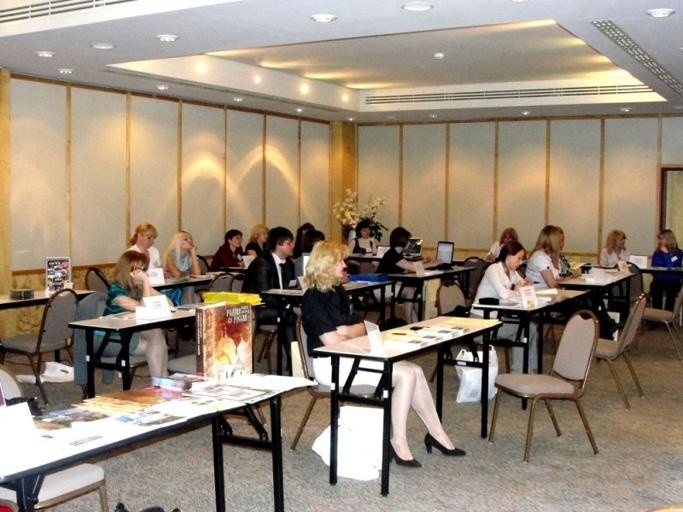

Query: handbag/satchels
311;406;384;481
455;345;499;402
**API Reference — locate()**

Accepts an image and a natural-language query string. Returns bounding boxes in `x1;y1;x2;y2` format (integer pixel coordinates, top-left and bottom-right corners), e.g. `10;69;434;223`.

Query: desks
262;281;396;375
348;253;382;276
0;373;319;511
639;266;683;328
452;257;527;299
388;266;477;322
472;288;590;412
552;269;637;361
313;315;504;499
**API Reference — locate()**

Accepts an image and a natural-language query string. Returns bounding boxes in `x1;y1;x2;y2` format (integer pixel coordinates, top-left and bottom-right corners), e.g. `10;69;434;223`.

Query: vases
346;231;356;250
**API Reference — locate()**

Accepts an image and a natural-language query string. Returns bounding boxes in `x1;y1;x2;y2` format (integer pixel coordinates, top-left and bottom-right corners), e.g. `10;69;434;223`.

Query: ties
279;263;288;289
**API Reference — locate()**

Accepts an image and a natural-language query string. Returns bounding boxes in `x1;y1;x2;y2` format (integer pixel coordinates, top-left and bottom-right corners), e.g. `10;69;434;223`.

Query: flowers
329;188;388;243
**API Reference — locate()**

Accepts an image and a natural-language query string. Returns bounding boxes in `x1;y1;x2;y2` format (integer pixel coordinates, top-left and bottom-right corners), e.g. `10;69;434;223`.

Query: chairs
375;246;390;257
594;292;649;409
642;283;683;358
462;257;486;297
0;370;109;512
488;310;602;464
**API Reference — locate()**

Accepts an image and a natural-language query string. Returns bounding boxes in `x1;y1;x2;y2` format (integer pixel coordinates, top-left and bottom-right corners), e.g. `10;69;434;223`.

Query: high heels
425;432;466;457
389;440;422;467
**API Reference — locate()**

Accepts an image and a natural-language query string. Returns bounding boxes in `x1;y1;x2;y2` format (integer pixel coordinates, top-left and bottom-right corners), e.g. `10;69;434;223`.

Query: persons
600;230;628;266
526;225;570;288
93;218;444;323
241;227;298;375
103;250;175;378
470;240;538;373
301;239;466;468
651;230;683;327
486;228;518;261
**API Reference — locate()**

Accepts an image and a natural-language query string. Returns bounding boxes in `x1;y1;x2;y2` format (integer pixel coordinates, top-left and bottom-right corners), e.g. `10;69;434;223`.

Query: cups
590;268;605;284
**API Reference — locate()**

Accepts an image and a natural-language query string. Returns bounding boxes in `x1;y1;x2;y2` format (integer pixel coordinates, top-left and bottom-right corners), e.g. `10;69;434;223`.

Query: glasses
133;265;149;272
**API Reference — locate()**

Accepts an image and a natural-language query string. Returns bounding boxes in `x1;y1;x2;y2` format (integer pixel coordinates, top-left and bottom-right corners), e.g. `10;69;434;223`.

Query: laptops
403;238;424;258
424;241;454;269
